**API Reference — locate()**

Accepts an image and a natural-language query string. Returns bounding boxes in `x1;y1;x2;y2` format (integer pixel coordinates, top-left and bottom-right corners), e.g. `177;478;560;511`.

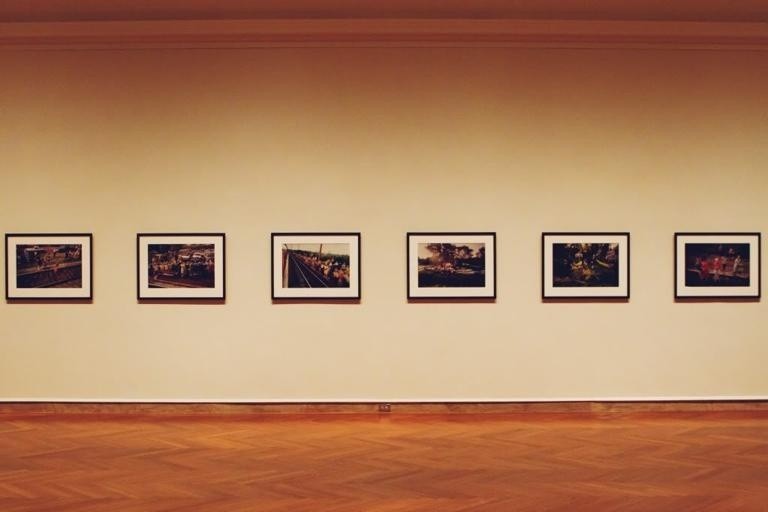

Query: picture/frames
540;231;630;301
673;231;762;300
4;231;94;302
270;232;362;302
405;231;496;301
136;232;227;303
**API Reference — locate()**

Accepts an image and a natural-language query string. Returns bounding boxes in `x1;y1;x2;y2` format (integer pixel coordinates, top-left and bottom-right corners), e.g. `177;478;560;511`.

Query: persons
694;255;742;280
